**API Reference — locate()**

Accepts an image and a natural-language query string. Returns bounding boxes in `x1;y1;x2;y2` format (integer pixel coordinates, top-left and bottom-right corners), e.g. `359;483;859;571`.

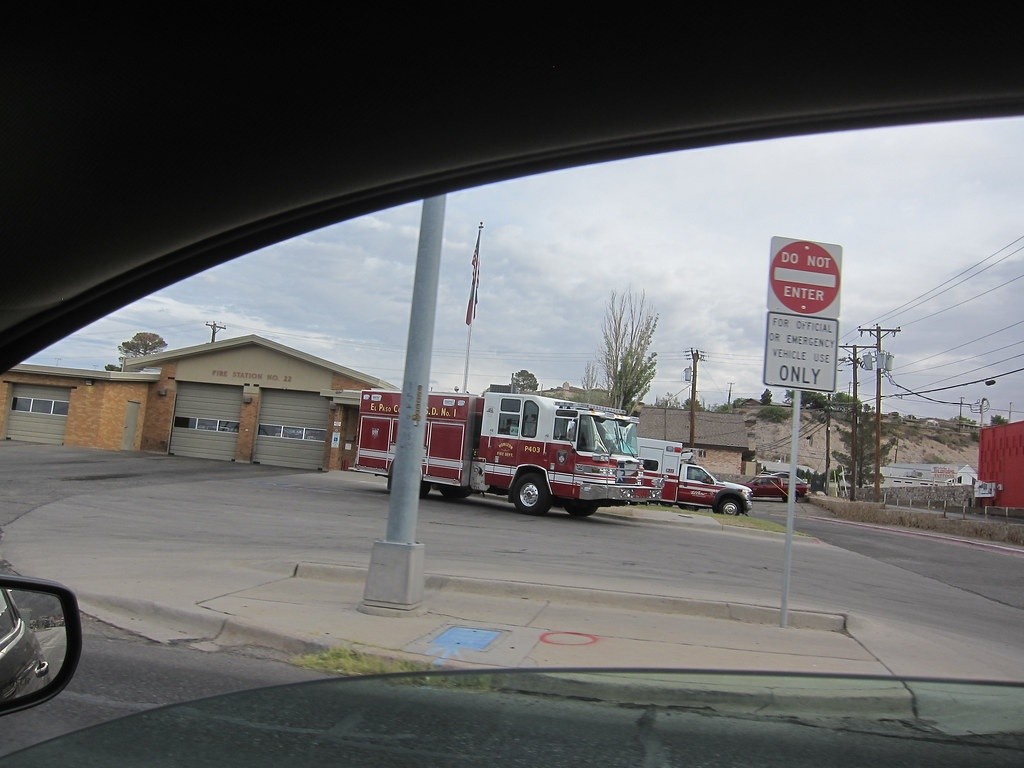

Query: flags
465;228;481;324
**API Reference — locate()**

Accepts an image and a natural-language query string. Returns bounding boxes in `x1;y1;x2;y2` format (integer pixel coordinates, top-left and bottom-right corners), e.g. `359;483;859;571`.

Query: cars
772;472;807;485
0;585;53;701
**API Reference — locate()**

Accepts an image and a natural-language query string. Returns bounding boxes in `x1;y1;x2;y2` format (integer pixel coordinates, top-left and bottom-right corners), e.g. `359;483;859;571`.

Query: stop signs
766;235;842;318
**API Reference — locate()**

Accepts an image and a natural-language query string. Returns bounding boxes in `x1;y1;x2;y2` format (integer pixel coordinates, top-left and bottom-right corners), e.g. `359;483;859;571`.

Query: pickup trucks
737;476;807;503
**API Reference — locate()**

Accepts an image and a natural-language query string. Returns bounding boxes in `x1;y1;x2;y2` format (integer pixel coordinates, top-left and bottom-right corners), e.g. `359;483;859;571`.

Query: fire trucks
349;386;669;517
636;436;753;517
